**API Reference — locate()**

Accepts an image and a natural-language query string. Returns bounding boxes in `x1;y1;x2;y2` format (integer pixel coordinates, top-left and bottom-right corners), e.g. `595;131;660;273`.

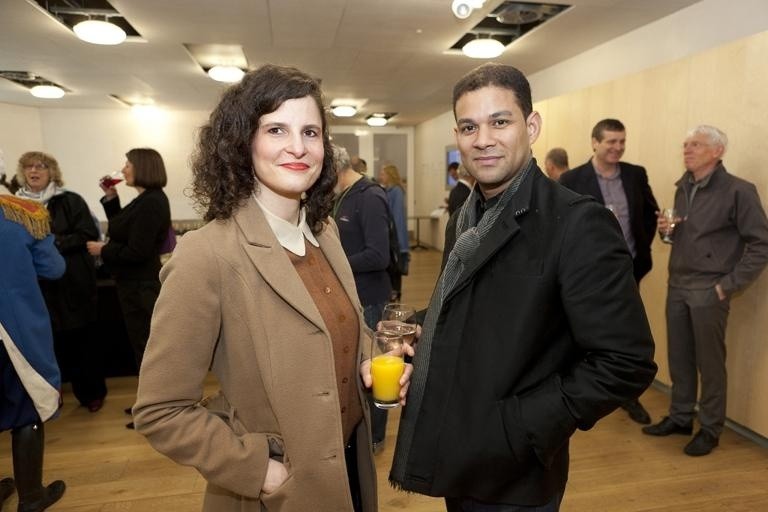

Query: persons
9;174;23;193
556;118;661;424
443;160;461;214
642;122;767;460
328;141;395;456
447;159;476;218
544;146;569;182
378;163;410;304
350;154;372;184
130;62;424;512
1;190;67;512
375;60;659;512
85;148;175;432
360;158;375;182
0;174;10;189
14;151;108;413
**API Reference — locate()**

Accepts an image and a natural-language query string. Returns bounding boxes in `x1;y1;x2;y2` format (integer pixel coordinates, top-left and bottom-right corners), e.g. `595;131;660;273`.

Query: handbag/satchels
159;225;177;256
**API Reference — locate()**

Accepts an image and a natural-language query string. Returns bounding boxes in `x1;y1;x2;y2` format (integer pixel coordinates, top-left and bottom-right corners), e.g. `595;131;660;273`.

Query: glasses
25;164;47;171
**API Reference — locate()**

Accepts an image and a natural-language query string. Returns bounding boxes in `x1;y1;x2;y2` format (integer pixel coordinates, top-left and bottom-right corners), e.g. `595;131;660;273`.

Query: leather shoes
624;399;651;424
641;415;692;436
682;427;721;457
87;386;108;413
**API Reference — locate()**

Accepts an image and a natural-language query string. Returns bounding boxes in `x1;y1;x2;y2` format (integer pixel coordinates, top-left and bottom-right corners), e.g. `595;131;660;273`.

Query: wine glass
661;209;679;244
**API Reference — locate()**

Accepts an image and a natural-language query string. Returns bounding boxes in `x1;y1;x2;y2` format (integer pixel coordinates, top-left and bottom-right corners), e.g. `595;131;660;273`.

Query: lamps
462;33;506;60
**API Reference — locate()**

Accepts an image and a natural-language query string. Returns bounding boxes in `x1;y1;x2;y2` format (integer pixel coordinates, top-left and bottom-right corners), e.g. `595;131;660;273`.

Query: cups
99;170;125;192
368;331;405;409
376;301;421;347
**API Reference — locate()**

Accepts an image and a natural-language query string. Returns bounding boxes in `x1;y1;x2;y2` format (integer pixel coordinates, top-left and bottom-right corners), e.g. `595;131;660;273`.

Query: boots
0;423;66;512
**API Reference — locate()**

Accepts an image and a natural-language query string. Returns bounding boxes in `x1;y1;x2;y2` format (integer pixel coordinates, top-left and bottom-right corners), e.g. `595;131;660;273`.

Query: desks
407;216;436;251
